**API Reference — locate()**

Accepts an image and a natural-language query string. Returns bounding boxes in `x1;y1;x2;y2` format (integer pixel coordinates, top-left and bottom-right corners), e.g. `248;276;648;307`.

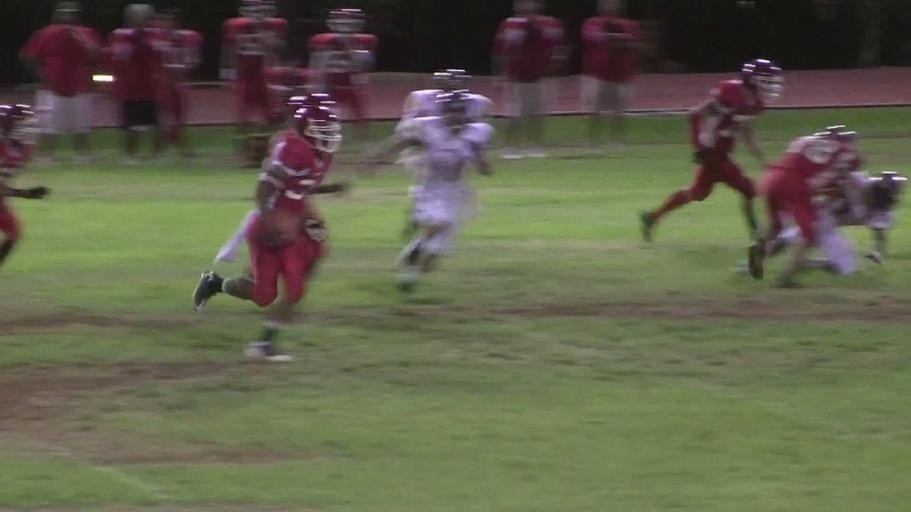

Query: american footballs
266;203;298;245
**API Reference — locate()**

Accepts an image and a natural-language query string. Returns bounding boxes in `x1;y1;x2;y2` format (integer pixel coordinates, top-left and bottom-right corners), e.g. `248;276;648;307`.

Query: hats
53;2;80;13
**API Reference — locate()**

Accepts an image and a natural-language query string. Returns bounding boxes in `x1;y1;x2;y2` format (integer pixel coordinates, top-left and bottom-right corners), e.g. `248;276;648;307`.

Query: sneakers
191;269;223;313
639;209;655;241
770;274;799;288
243;336;294;366
749;239;765;279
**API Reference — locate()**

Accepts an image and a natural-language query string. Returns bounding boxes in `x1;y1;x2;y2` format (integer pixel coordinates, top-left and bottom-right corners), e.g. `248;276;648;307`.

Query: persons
491;0;568;160
144;3;203;164
0;101;54;267
18;0;108;169
355;88;498;297
390;68;495;247
217;0;301;171
632;52;789;245
744;121;867;293
182;91;349;368
305;5;385;162
92;0;183;168
572;0;648;158
731;167;907;285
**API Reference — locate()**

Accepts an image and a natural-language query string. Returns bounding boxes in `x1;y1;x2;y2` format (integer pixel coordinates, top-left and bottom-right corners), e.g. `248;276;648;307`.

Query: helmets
0;103;39;146
738;57;784;106
818;122;857;146
867;167;904;214
435;69;470;91
123;4;157;28
325;8;368;33
293;104;344;158
237;4;277;19
438;89;473;133
